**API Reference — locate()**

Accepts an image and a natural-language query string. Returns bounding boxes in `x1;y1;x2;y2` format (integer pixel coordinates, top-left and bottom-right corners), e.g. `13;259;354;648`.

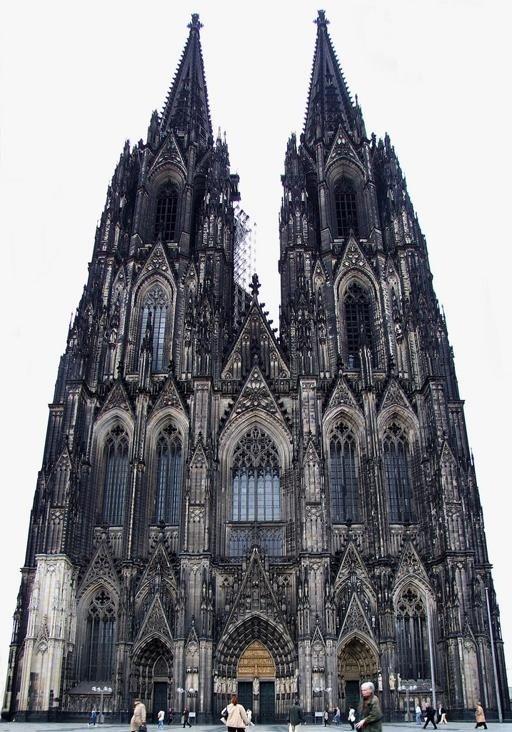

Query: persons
324;709;332;728
347;705;356;730
334;707;341;725
130;698;147;732
167;707;175;725
439;704;448;723
156;707;166;728
354;681;384;732
474;701;487;730
225;695;251;732
286;701;306;732
88;705;97;726
182;706;192;728
422;702;438;730
415;703;422;725
435;704;443;724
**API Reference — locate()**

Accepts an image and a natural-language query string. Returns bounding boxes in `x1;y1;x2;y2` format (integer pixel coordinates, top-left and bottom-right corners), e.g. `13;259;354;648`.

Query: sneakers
156;725;192;729
416;723;488;730
88;723;98;728
323;724;355;731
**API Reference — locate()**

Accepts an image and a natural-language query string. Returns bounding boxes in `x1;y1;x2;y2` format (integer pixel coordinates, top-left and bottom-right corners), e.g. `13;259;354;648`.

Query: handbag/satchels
222;706;228;721
139;724;147;732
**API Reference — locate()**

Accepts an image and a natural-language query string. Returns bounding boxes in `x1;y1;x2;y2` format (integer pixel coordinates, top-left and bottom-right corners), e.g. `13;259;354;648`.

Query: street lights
397;685;418;721
177;688;195;725
92;685;113;723
315;687;333;724
